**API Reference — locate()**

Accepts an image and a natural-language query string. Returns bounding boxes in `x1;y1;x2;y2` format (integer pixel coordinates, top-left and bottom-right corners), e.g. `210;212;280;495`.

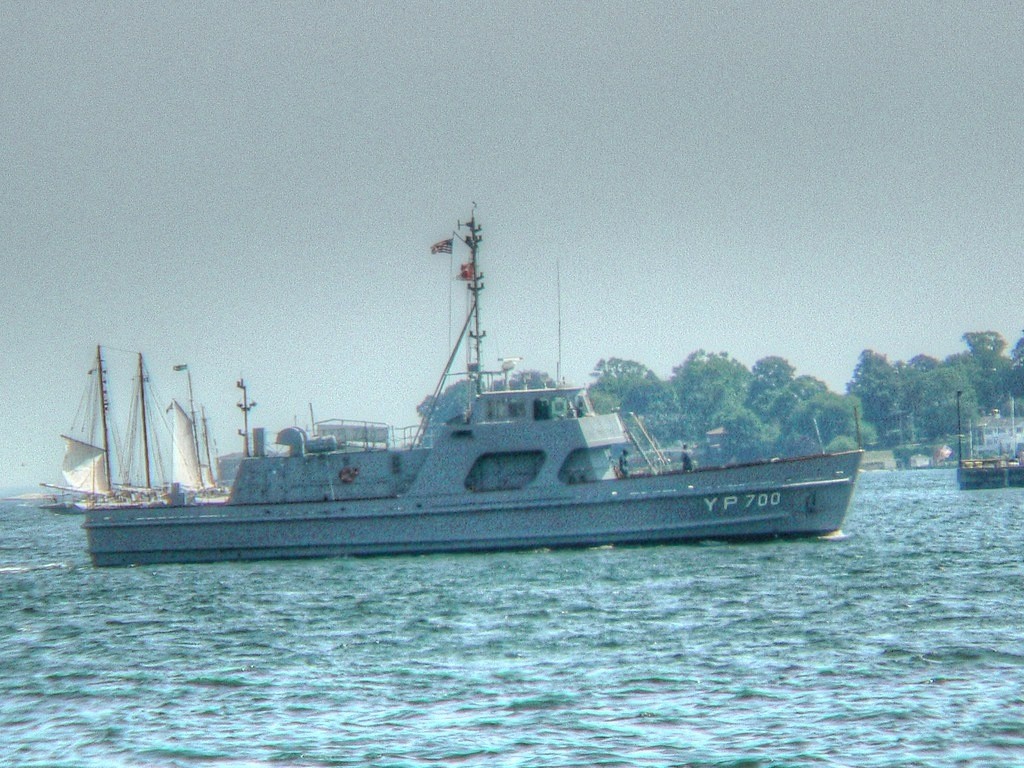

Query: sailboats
38;344;232;515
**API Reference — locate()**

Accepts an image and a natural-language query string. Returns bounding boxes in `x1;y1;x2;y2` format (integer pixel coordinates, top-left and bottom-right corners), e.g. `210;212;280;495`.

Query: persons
534;398;549;420
680;443;694;474
576;400;584;416
619;448;630;478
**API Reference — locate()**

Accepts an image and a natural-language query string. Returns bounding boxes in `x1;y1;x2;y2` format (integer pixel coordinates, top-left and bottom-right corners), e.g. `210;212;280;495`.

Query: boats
80;200;866;569
956;441;1024;490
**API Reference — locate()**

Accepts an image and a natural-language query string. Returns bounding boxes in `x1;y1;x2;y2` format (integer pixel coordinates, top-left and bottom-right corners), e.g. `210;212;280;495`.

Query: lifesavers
339;466;358;484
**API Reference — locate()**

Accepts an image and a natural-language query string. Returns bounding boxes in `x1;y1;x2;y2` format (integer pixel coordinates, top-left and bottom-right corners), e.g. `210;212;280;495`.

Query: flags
430;239;452;255
